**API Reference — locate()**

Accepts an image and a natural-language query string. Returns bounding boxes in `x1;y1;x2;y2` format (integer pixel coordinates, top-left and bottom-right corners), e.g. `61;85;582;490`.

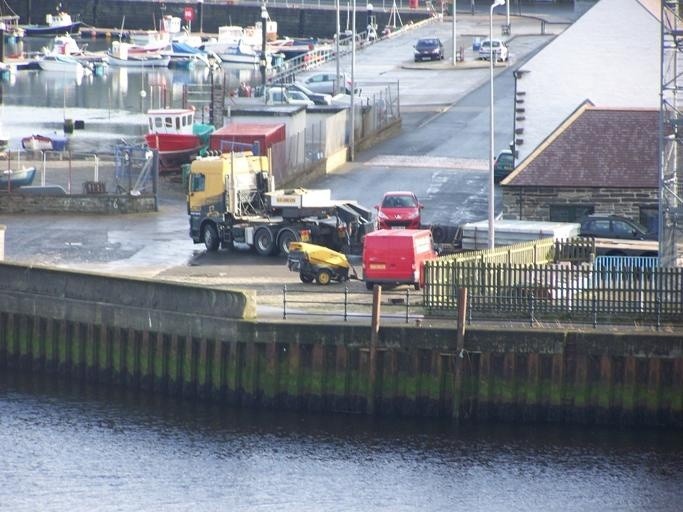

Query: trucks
185;149;375;259
224;86;316;107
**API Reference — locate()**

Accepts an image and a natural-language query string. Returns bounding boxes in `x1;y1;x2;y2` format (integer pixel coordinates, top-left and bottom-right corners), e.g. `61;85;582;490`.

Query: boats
20;134;71;152
144;107;216;159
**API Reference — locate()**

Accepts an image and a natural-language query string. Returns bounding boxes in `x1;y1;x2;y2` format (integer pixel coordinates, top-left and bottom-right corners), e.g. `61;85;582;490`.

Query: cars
413;38;444;61
270;73;358;105
373;190;424;230
493;148;515;183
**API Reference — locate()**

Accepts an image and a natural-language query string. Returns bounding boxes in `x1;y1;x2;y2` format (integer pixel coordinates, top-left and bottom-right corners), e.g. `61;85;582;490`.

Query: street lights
367;3;373;42
488;0;506;250
259;9;267;86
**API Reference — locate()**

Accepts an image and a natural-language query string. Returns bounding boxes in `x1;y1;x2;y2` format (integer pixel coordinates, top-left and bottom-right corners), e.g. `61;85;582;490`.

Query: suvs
579;212;659;257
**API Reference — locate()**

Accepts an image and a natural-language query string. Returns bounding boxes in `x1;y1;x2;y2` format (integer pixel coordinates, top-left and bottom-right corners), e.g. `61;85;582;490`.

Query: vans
363;228;439;292
479;39;509;63
